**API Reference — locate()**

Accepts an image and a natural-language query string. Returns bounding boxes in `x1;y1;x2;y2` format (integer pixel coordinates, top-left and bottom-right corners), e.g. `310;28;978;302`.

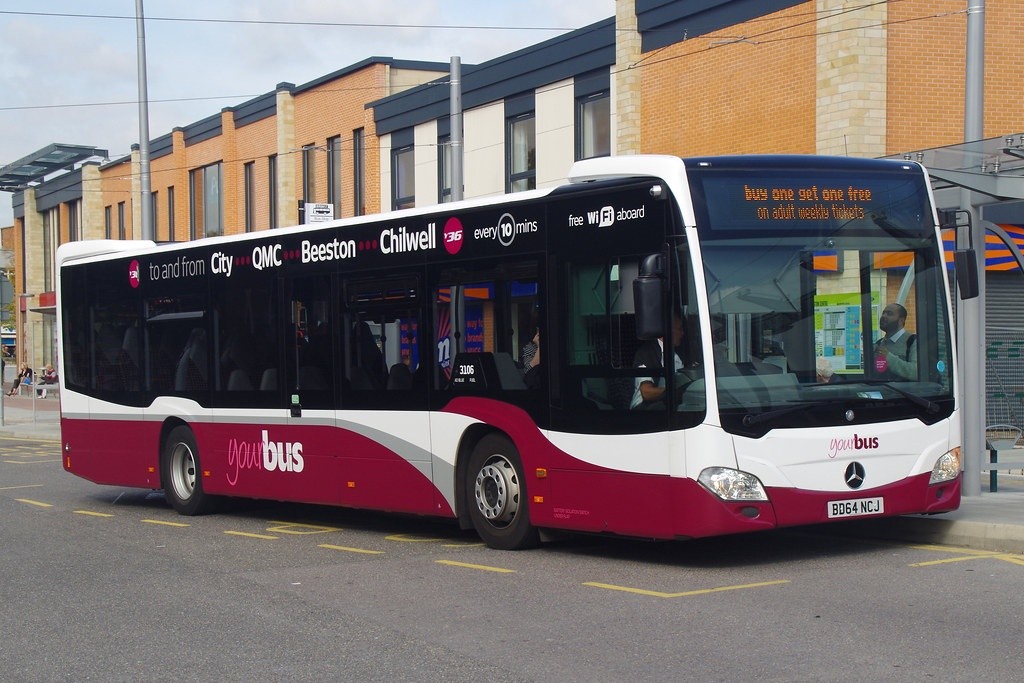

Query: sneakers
37;396;46;399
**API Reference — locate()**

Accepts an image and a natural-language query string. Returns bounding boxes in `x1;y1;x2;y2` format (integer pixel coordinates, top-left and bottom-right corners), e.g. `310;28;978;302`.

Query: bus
51;153;981;552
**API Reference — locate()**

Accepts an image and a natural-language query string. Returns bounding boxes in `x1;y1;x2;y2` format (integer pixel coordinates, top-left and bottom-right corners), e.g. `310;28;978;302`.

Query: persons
7;362;32;395
874;304;918;380
630;317;684;410
522;310;539;375
37;365;57;398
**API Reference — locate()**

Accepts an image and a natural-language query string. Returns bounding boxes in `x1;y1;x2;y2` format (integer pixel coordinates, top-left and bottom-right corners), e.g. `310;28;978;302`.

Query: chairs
92;322;415;393
593;314;642;411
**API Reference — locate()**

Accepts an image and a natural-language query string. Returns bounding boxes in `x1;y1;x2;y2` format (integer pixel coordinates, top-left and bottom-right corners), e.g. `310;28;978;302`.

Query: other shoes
6;392;17;396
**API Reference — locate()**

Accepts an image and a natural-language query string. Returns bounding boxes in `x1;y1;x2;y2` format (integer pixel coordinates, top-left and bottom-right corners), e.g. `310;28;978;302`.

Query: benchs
35;384;59;398
19;383;29;396
